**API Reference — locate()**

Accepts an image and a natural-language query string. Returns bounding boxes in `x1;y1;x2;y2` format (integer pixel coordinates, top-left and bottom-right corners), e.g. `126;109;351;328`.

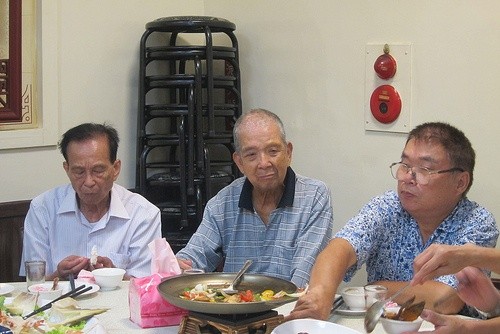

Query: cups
364;285;389;310
25;261;46;292
341;286;365;312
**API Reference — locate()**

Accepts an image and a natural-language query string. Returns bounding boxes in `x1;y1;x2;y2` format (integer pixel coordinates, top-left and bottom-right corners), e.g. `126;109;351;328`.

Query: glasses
389;162;463;185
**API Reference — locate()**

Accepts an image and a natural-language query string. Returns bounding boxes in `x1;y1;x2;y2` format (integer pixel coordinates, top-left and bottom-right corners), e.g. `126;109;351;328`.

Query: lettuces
0;296;88;334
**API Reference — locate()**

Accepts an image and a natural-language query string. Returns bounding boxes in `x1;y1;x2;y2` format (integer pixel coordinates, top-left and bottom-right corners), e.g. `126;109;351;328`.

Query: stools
135;16;243;254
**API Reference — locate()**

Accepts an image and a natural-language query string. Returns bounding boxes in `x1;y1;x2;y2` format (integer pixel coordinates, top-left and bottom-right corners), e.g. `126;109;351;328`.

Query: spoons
216;260;253;294
364;283;411;333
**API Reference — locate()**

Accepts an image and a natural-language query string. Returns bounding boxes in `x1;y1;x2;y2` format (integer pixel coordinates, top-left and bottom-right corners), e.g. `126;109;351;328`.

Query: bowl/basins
93;267;126;290
380;306;423;333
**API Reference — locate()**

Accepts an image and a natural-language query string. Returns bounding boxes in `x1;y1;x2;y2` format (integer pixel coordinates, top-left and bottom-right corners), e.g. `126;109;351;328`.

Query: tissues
129;238;189;329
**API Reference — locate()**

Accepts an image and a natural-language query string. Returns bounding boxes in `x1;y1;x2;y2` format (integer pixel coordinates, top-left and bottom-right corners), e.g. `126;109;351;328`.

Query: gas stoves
176;308;283;334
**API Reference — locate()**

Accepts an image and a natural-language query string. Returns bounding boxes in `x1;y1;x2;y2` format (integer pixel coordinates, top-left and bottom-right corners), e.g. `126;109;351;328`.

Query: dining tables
0;281;436;334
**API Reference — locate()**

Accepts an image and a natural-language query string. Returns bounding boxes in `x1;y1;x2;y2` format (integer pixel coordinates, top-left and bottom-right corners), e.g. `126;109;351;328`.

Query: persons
19;120;162;280
399;242;500;334
175;108;334;288
285;122;500;321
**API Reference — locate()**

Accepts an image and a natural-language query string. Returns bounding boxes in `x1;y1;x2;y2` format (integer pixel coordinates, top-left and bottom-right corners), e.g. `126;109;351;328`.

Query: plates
335;303;366;316
28;280;100;297
0;282;16;296
156;271;296;315
271;319;359;334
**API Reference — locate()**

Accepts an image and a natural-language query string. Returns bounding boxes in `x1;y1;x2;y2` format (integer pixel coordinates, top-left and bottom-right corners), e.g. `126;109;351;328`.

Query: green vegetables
182;286;262;301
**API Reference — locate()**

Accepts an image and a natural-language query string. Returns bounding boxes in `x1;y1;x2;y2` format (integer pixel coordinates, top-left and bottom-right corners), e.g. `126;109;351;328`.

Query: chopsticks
23;285;92;320
331;297;344;312
